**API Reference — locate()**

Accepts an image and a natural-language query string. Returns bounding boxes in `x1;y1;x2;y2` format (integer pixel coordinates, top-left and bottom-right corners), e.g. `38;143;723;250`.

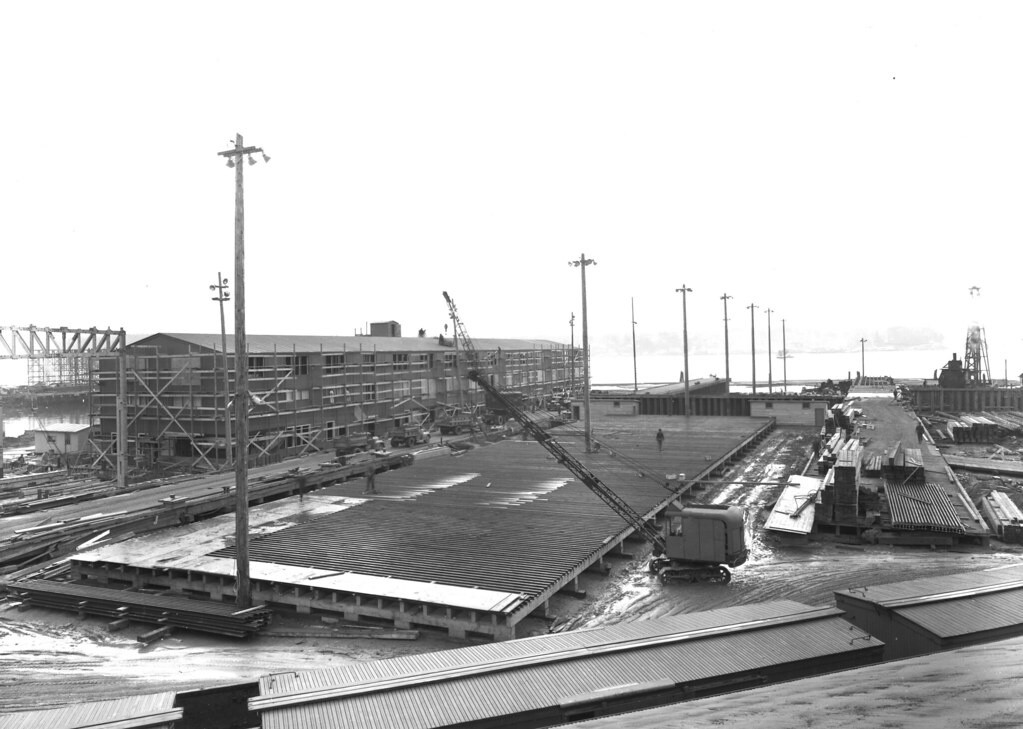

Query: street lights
720;292;733;395
568;253;597;452
860;337;867;380
210;272;232;465
746;303;759;393
569;312;575;392
676;284;692;417
218;146;270;608
764;308;774;393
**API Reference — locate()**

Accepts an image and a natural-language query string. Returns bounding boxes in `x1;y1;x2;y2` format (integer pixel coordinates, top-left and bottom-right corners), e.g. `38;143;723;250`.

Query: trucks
333;431;385;457
435;412;483;435
546;386;571;411
387;423;431;448
481;392;524;425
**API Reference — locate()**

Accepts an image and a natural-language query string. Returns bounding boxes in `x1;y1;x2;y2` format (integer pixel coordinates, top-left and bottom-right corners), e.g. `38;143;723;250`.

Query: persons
894;389;897;398
366;463;375;490
656;428;664;450
42;451;48;464
19;453;24;463
916;422;924;444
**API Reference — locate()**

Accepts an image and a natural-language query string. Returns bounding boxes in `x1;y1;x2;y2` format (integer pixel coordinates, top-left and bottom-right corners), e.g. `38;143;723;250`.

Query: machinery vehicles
466;369;751;587
